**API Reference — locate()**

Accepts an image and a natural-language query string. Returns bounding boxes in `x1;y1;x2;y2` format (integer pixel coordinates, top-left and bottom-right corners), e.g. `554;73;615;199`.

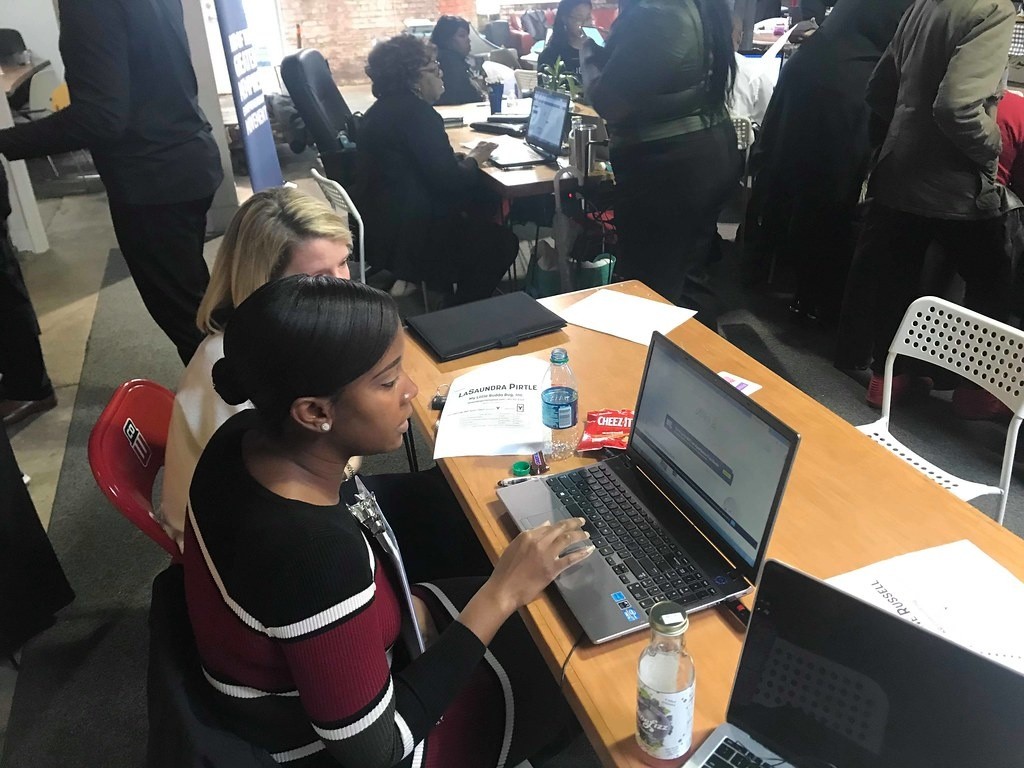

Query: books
405;289;569;363
469;111;532;136
442;117;463;128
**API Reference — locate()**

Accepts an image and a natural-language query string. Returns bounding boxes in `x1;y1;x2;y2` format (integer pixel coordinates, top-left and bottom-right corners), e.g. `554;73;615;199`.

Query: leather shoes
0;393;54;427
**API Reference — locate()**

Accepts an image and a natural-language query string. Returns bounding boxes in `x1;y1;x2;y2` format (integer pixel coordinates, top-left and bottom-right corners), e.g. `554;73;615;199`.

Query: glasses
415;61;441;76
570;15;592;25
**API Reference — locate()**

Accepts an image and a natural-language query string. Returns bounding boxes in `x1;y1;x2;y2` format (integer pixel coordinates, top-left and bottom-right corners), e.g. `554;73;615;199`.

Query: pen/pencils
497;473;555;487
501;165;537;170
476;105;490;107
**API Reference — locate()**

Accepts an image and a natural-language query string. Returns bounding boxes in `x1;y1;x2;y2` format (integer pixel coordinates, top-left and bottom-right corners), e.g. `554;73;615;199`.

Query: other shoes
790;300;846;328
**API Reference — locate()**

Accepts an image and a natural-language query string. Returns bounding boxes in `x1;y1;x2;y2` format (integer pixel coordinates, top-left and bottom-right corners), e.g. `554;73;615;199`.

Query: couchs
504;5;620;70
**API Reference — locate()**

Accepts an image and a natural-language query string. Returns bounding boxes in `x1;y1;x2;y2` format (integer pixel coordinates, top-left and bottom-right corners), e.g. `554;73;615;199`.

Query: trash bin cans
523;252;616;299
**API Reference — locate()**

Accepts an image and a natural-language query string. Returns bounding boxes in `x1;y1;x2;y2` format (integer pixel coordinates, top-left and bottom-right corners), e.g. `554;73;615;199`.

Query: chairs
852;296;1024;525
86;379;181;556
514;69;541;100
537;72;581;100
145;555;282;768
280;47;506;314
481;60;518;101
489;49;524;70
716;116;758;225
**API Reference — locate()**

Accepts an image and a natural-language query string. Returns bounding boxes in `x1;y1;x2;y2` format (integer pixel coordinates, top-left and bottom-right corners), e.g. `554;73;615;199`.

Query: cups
486;84;504;115
569;130;575;166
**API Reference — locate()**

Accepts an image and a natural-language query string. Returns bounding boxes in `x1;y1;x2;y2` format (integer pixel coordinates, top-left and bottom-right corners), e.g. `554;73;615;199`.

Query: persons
537;0;600;89
995;90;1024;189
865;0;1024;421
0;0;225;368
429;14;487;106
580;0;740;297
182;274;595;768
159;186;353;554
348;35;519;310
749;0;916;325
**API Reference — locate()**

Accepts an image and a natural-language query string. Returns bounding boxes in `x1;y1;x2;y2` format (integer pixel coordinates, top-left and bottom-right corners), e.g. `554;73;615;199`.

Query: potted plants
533;54;582;144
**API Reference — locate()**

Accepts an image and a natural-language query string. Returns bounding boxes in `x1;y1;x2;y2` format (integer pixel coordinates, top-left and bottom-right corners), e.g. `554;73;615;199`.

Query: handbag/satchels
522;239;616;297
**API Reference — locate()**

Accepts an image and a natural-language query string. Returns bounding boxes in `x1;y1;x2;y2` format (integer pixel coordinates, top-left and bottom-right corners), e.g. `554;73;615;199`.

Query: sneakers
953;389;1015;419
867;371;932;407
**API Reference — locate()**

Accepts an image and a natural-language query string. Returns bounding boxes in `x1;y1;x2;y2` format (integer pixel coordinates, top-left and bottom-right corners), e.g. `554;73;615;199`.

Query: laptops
679;558;1024;768
486;87;572;168
495;331;802;646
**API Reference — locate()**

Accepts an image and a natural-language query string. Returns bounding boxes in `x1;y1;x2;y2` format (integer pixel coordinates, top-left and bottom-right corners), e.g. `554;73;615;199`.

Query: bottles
635;602;696;768
574;124;597;174
539;348;579;461
571;116;583;129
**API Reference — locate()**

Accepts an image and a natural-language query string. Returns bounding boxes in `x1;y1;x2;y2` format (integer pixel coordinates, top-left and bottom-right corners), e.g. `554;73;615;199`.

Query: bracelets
346;463;355;480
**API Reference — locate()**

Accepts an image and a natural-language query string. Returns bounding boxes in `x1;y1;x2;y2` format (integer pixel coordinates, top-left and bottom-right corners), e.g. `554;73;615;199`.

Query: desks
399;280;1024;768
0;59;52;253
432;97;753;294
